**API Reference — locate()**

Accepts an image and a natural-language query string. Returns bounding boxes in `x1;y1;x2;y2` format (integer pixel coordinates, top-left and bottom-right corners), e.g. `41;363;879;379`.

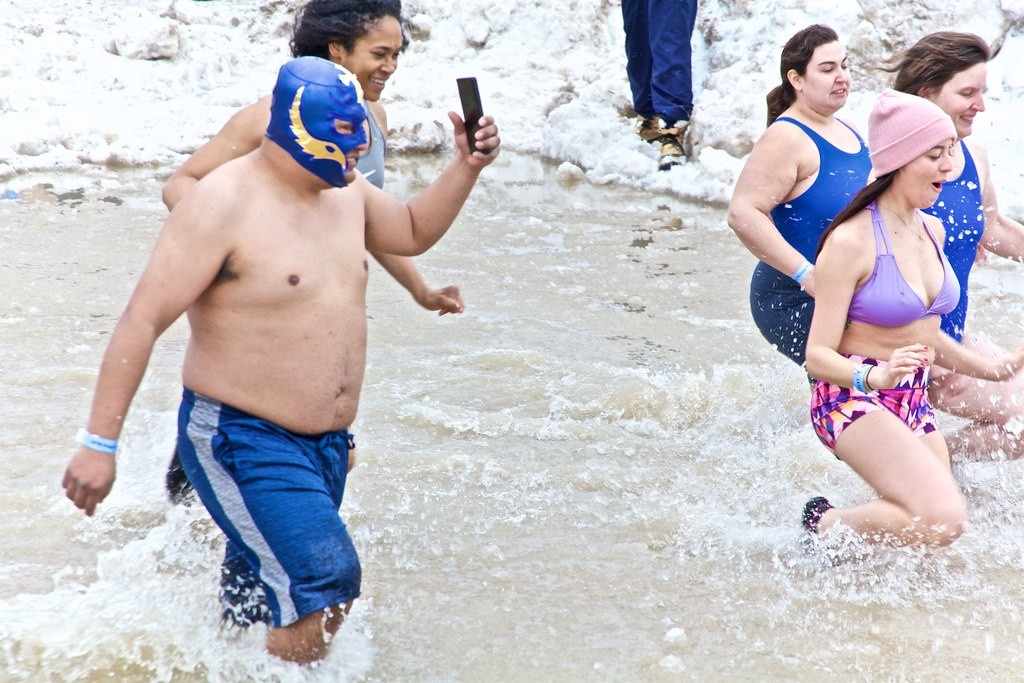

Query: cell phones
456;77;491;155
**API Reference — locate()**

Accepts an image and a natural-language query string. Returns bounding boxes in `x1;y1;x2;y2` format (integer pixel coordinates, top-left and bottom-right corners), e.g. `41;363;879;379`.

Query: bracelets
789;259;814;284
852;362;876;396
74;426;120;455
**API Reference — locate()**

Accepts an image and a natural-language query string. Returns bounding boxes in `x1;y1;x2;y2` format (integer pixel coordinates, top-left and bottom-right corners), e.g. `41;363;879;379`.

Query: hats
867;88;957;180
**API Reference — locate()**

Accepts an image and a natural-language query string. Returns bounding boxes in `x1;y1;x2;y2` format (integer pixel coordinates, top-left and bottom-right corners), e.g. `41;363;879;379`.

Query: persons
158;0;465;513
892;29;1023;466
61;52;503;664
622;0;701;172
797;84;1023;564
726;21;877;396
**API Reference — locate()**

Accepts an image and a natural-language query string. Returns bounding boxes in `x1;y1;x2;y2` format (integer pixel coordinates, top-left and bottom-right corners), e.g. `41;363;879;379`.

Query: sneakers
804;496;834;538
635;116;663;143
658;126;686;171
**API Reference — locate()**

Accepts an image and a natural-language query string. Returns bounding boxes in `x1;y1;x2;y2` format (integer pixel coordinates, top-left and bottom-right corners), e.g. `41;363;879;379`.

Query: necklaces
876;197;926;244
